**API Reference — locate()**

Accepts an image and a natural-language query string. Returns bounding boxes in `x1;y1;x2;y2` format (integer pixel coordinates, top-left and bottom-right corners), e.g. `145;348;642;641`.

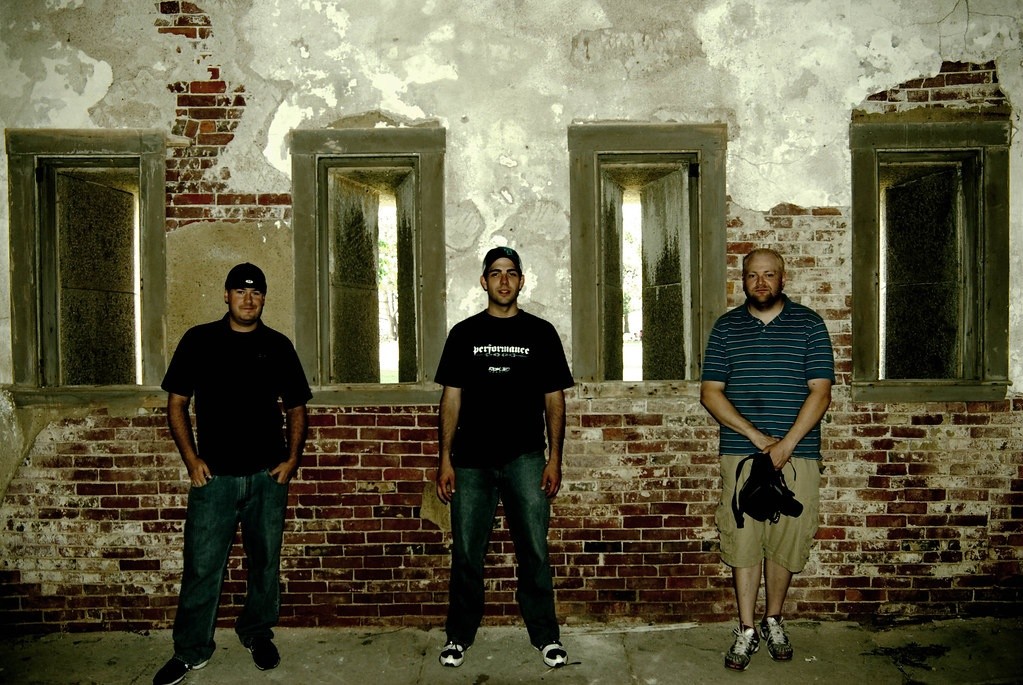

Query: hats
482;247;520;275
224;263;266;289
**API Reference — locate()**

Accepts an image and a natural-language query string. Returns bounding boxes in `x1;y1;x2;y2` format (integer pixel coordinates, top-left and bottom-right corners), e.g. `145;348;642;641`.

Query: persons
436;247;576;668
697;248;835;668
150;262;314;685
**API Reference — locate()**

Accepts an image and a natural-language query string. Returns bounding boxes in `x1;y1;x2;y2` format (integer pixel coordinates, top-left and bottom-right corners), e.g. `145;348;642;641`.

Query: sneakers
530;637;569;668
725;623;759;670
439;640;471;667
759;614;793;661
152;656;208;685
251;637;280;670
